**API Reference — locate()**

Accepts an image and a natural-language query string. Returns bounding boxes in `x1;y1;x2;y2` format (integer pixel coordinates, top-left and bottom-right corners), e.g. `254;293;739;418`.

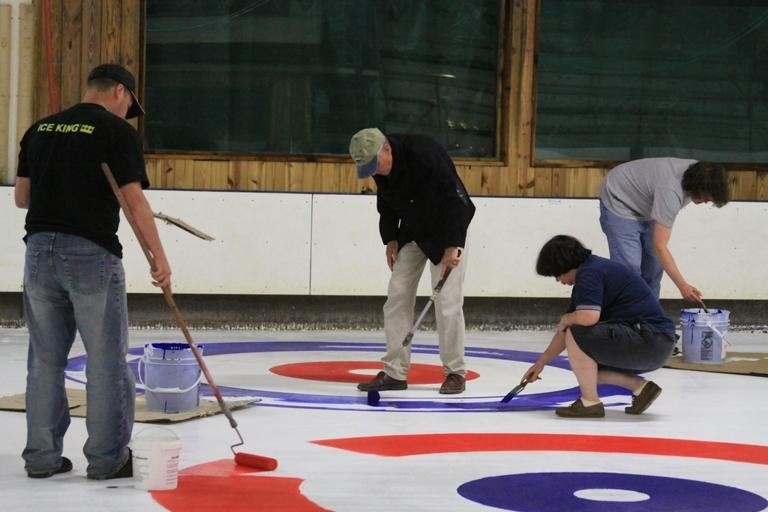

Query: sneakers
106;449;133;481
624;380;663;415
556;398;606;418
25;457;73;478
438;373;466;394
358;370;407;392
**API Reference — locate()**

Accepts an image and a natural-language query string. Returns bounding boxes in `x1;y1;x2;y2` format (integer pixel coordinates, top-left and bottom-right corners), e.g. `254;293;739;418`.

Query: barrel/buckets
129;426;181;490
137;341;203;412
679;307;730;362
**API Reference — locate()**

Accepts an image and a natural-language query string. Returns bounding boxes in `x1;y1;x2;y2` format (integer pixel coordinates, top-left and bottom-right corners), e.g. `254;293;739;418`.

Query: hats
90;61;147;121
349;127;387;180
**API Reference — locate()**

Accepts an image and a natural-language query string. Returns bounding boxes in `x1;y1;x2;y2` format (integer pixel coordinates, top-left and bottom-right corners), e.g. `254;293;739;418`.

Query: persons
349;129;476;394
14;63;174;480
522;235;677;417
600;157;730;306
